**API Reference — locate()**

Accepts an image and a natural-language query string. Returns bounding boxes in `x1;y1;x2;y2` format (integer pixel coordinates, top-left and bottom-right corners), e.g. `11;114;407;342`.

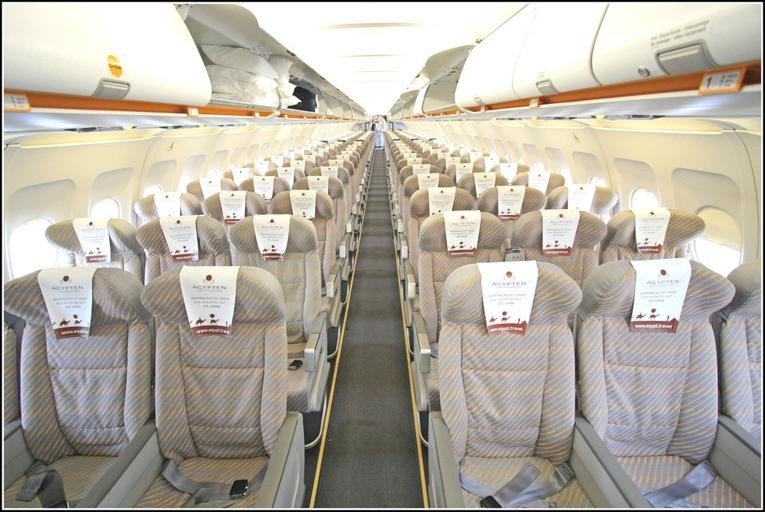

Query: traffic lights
201;43;301;107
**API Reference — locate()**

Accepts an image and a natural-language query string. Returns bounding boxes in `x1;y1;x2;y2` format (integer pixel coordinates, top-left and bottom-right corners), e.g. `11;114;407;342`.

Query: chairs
384;129;761;509
5;127;375;507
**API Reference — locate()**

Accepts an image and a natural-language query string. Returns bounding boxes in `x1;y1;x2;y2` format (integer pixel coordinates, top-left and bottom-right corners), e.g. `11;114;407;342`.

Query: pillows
201;43;301;107
